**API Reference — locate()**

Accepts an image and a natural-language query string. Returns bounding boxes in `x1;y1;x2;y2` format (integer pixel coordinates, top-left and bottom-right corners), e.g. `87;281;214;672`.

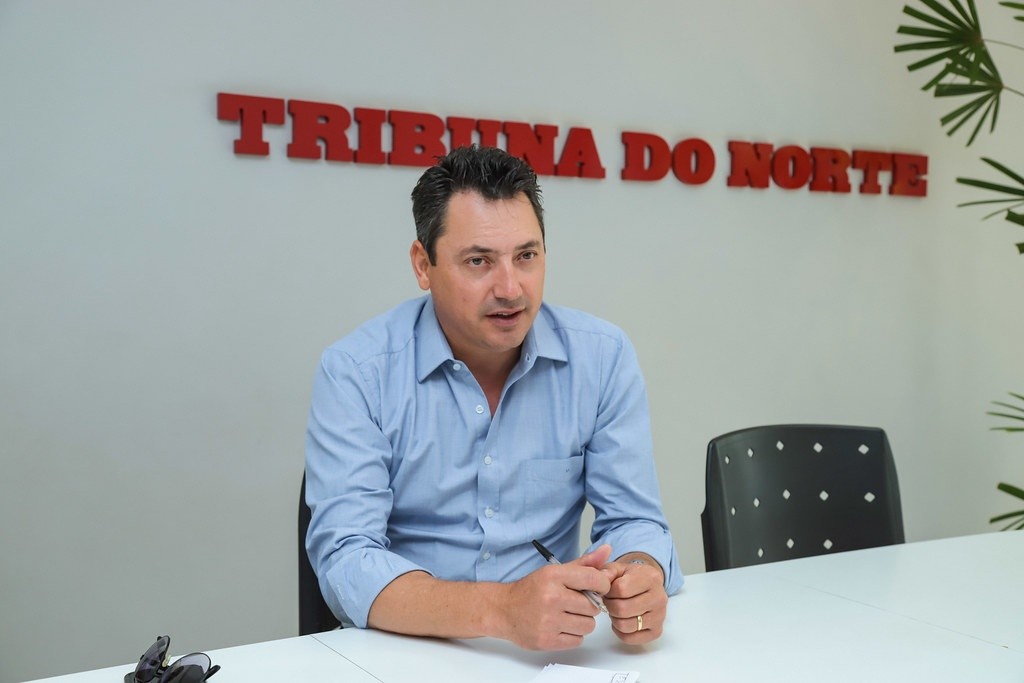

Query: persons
305;143;685;650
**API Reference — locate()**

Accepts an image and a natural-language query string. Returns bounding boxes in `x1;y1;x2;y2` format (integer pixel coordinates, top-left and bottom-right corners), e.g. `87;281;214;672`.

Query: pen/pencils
531;539;609;614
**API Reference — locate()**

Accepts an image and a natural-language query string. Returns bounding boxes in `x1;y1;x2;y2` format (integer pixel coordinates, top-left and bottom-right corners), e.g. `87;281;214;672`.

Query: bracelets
629;559;643;564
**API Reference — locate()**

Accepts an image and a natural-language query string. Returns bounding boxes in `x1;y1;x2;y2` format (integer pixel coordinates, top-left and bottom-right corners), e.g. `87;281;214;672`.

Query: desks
30;529;1024;683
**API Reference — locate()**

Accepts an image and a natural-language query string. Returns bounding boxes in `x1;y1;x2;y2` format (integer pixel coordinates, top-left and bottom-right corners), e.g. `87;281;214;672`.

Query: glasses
124;636;221;683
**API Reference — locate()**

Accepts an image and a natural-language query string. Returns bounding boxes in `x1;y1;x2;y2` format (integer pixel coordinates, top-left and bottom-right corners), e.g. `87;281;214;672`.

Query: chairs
700;424;905;572
297;471;343;636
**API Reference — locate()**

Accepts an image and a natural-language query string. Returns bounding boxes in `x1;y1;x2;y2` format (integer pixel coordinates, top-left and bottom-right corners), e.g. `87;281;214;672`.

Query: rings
638;616;642;631
